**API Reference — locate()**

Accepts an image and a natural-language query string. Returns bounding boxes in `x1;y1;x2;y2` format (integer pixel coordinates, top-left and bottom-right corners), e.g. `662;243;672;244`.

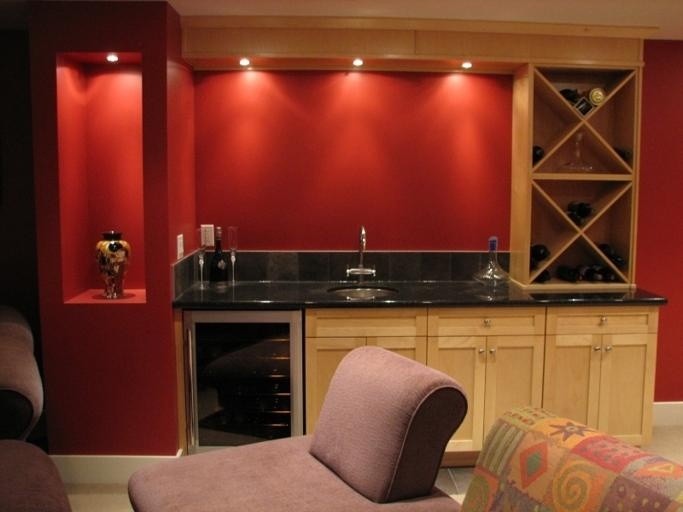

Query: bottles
529;242;627;286
211;225;227;284
471;235;511;290
564;201;597;224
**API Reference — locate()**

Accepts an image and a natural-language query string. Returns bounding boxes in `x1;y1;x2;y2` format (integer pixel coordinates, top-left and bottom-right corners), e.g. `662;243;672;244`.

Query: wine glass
226;225;240;285
193;227;209;289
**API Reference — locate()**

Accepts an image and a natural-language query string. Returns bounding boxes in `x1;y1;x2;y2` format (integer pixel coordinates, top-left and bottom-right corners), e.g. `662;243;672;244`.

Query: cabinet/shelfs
509;62;642;294
303;306;667;471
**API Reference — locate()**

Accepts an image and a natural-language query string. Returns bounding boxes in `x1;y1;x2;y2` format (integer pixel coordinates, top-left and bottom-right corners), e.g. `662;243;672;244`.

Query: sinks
328;285;398;300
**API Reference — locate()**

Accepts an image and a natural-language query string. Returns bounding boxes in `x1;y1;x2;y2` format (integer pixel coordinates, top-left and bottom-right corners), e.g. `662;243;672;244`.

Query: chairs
125;345;470;512
459;403;683;512
1;287;79;512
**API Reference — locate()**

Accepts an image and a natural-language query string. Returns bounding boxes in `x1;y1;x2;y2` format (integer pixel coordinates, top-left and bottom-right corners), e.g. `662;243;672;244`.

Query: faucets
359;225;367;265
